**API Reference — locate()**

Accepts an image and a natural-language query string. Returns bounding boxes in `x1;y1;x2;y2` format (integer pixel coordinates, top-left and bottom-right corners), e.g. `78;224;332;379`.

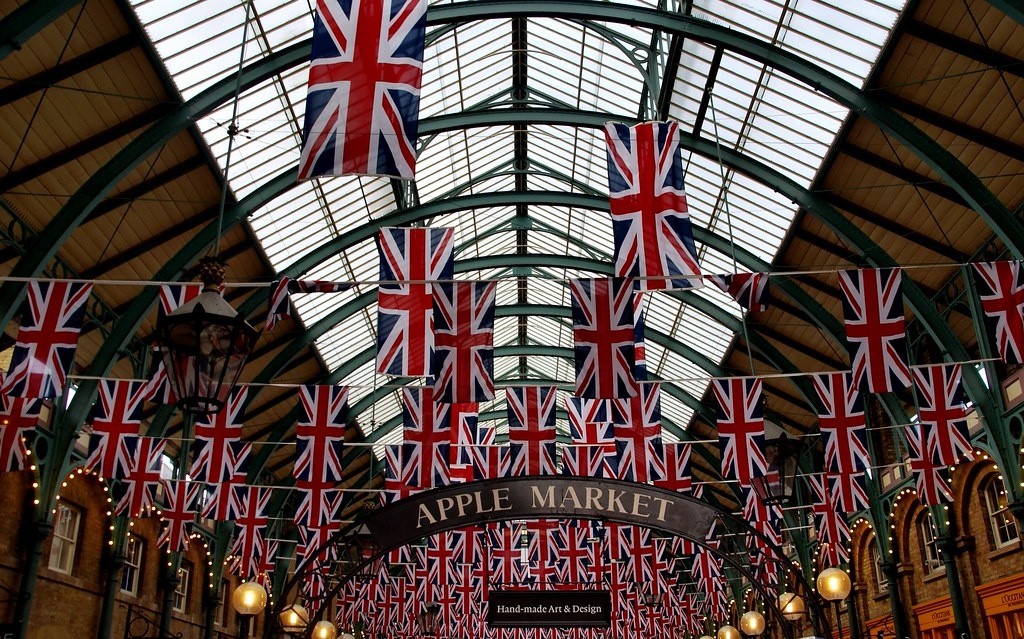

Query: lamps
416;603;441;635
639;582;666;607
705;87;801;505
155;1;264;414
233;583;356;639
346;326;386;580
695;568;852;639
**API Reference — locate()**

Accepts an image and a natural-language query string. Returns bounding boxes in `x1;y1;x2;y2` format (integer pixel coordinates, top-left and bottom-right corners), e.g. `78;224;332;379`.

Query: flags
0;226;1024;638
599;120;705;293
292;0;429;179
